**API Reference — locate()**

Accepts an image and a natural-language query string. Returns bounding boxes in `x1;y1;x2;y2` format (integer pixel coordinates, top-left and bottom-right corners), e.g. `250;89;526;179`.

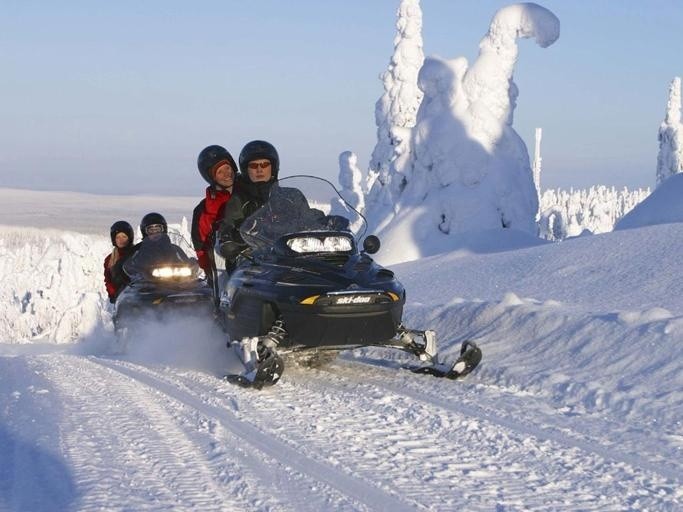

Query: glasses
144;223;164;237
246;161;272;170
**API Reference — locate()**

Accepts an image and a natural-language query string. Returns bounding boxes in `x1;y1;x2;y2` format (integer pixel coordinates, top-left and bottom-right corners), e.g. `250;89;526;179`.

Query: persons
215;140;349;277
104;221;136;305
120;212;198;283
190;144;240;283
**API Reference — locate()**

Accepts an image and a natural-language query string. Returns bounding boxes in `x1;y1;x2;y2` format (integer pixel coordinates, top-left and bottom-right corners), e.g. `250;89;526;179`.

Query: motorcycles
105;225;233;353
199;171;485;391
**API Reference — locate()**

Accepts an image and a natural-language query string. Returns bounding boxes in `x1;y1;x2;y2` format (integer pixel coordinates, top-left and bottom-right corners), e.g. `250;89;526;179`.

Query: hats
204;159;231;182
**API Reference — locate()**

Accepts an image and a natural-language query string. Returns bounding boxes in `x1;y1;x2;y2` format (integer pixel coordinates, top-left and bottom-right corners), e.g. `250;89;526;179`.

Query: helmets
139;212;167;239
109;220;135;247
237;138;281;177
195;143;239;187
